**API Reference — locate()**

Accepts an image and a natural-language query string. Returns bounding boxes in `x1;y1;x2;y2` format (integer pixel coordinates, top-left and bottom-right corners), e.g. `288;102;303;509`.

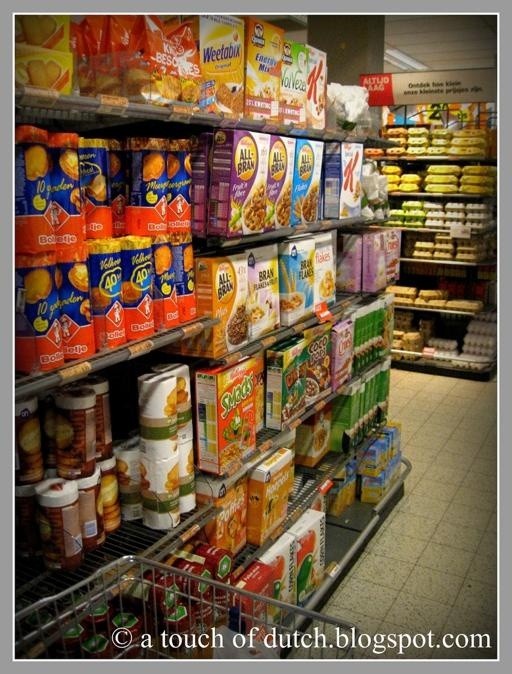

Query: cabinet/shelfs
17;82;223;660
374;121;497;381
215;117;412;660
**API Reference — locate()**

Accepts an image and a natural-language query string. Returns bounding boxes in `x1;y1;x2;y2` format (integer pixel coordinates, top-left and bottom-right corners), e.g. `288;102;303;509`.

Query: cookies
40;16;56;34
21;15;42;44
45;60;61;85
228;305;248;345
28;60;47;88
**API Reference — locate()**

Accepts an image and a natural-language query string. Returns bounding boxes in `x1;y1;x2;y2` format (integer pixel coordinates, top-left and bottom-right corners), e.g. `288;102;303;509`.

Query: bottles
34;478;83;569
15;396;45;485
54;388;96;480
76;464;106;551
82;378;112;462
96;455;122;532
39;390;55;468
15;485;36;558
